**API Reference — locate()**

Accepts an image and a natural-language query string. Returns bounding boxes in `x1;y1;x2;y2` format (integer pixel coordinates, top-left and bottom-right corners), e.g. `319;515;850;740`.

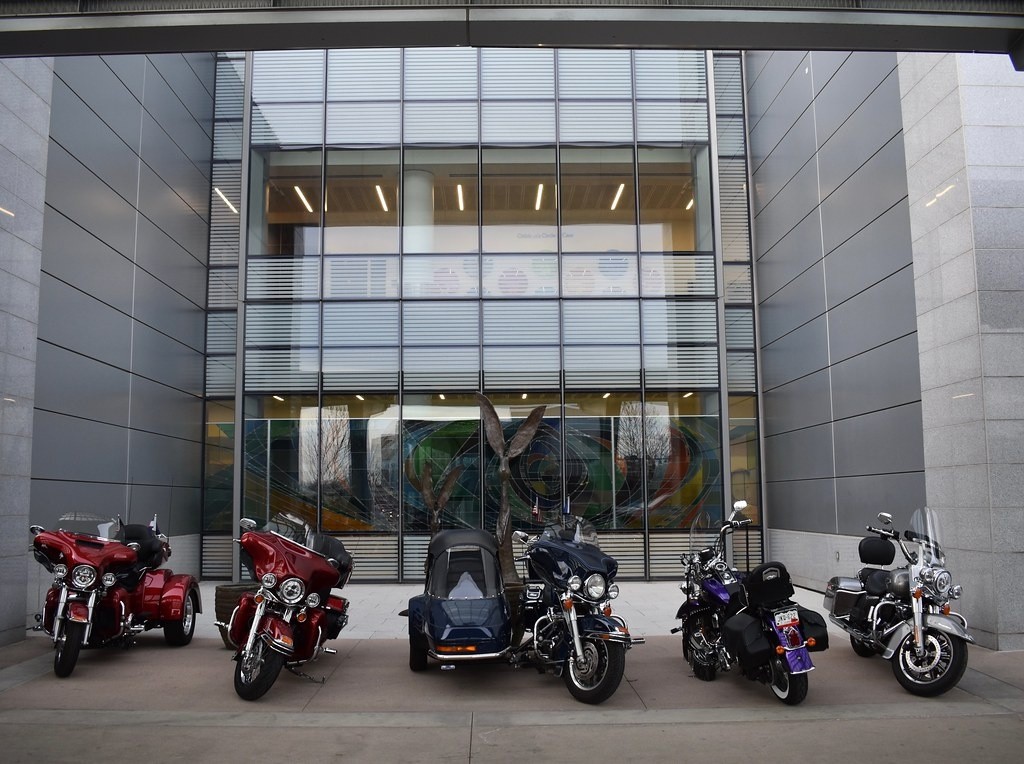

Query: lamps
610;184;625;210
683;392;693;398
685;199;693;210
603;393;610;398
356;395;365;400
521;394;527;399
273;395;284;401
375;185;389;211
535;184;543;210
439;394;445;399
293;184;313;213
457;184;464;210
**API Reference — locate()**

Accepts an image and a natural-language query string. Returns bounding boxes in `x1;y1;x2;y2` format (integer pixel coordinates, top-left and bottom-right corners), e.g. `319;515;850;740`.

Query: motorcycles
670;500;830;704
395;494;646;706
25;508;203;678
212;512;355;702
823;505;976;695
